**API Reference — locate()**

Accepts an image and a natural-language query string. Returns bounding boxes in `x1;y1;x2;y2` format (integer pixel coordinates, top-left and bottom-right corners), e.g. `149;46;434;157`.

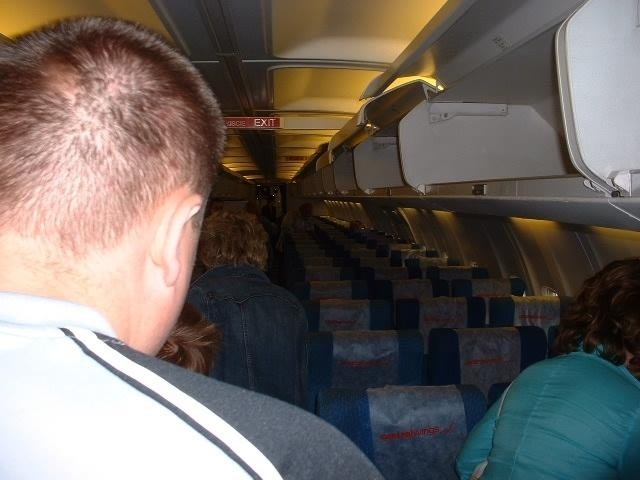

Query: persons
281;203;311;235
157;304;217;376
0;17;383;480
184;206;310;407
453;257;640;479
